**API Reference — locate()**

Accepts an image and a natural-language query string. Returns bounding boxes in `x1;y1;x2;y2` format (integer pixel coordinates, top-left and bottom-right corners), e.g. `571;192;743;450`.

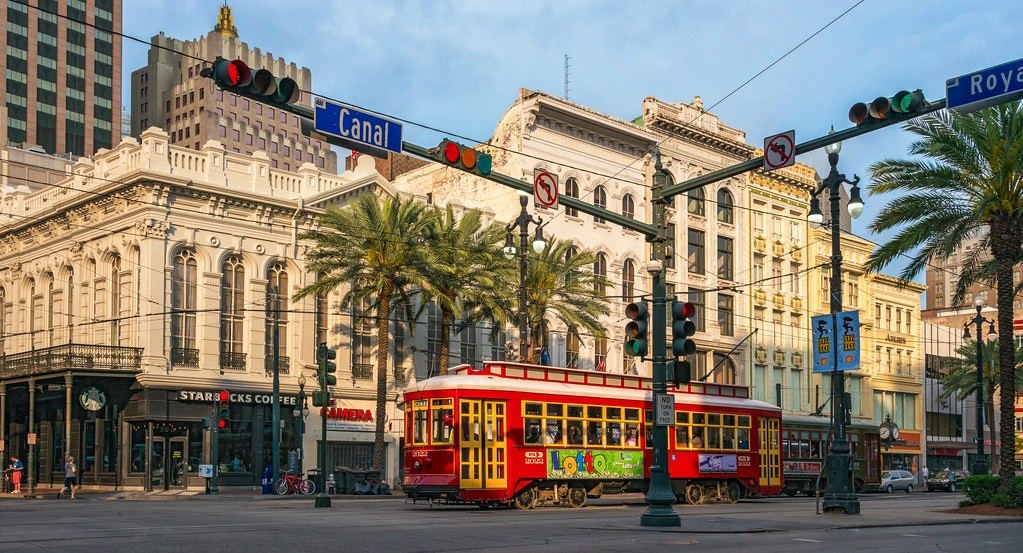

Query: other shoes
71;497;76;500
56;493;60;499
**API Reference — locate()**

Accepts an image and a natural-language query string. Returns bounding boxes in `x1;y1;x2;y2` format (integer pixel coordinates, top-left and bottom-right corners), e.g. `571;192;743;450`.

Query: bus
779;413;882;495
395;358;785;513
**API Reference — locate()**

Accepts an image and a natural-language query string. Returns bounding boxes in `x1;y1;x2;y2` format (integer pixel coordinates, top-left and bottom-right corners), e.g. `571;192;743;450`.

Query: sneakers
11;490;21;494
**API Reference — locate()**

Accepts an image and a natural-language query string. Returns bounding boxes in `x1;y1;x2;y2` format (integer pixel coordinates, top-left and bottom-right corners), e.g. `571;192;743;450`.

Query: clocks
879;427;891;440
892;427;899;439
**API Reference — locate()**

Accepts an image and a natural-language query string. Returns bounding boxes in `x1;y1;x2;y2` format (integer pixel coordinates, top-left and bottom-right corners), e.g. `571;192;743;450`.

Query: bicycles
273;468;316;495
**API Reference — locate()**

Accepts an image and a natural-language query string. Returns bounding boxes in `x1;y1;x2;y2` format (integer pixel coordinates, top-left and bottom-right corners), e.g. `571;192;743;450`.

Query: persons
57;456;79;500
8;454;24;494
681;425;749;449
921;465;929;487
526;422;581;445
624;426;636;446
806;441;818;458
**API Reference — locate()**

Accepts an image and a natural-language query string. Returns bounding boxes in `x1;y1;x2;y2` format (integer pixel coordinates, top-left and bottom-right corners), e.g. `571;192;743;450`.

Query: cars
873;470;915;494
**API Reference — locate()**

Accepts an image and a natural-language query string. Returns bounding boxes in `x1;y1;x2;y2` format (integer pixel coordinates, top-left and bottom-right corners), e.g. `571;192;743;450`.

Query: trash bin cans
307;468;322;493
262;466;273;493
332;465;379;494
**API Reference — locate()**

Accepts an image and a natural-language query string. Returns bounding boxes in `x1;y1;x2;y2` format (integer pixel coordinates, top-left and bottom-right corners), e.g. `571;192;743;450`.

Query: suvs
926;469;972;493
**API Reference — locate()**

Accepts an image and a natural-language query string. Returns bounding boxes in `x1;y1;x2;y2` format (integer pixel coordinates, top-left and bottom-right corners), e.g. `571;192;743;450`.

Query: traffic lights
210;58;300;106
671;300;696;357
624;302;649;356
218;390;231;430
667;359;692;385
849;89;924;125
317;345;337;386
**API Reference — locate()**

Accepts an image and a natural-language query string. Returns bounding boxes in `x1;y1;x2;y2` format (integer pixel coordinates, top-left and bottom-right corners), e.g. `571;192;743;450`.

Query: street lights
502;186;546;363
960;294;999;476
806;126;865;517
290;371;310;475
